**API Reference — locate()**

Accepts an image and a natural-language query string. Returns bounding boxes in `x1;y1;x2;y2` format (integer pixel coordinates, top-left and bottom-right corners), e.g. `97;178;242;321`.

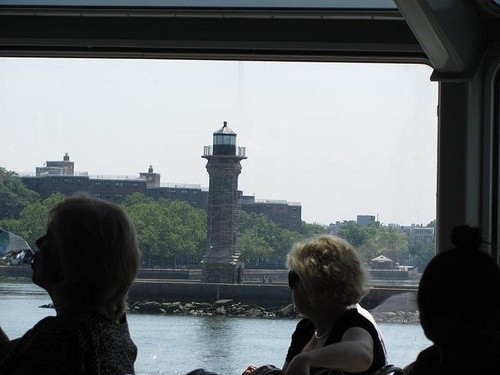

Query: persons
243;235;387;375
0;197;139;375
393;247;500;375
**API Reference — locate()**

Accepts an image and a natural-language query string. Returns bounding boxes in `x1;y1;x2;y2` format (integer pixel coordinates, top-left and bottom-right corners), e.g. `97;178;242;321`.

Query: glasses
288;270;299;286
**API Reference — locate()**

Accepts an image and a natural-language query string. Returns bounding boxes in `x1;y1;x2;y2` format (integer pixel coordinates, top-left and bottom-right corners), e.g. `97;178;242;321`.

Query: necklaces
314;328;329;339
